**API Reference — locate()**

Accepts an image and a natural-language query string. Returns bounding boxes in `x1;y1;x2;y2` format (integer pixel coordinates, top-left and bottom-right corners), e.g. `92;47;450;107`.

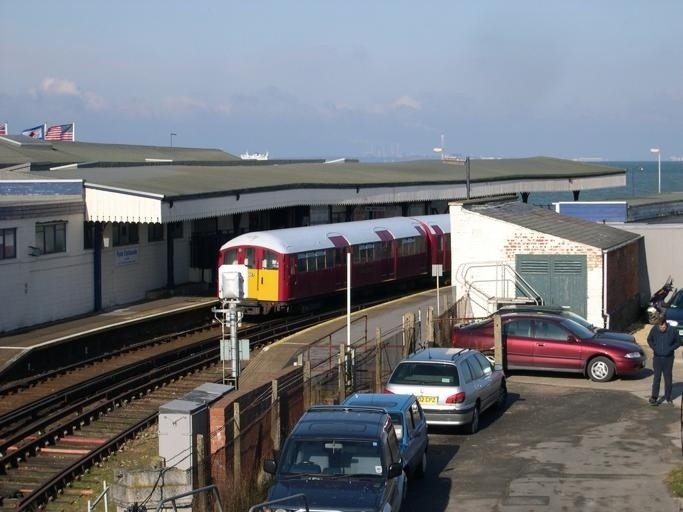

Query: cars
663;288;683;344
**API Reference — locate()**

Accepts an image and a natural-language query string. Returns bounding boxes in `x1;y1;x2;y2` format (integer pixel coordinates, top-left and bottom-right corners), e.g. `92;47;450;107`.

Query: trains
216;213;451;323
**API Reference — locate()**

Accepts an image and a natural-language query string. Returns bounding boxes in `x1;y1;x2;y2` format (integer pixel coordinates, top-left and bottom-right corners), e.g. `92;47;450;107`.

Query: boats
241;150;271;160
437;133;502;160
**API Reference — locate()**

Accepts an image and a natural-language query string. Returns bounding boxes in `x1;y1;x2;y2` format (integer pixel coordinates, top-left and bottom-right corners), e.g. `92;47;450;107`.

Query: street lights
650;148;661;193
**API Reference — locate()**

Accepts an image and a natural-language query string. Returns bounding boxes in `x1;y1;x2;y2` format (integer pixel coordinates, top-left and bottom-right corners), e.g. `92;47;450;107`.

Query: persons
646;317;682;404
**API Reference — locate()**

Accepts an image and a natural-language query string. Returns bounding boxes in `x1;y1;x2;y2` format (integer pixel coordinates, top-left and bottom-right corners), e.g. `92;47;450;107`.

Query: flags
21;124;43;140
0;120;8;135
45;124;73;141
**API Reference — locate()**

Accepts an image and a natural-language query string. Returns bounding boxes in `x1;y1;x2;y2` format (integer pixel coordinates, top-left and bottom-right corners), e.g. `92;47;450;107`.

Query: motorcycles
643;275;677;325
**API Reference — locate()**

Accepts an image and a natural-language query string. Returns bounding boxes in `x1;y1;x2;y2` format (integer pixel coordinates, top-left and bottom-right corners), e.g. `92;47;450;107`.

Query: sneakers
649;397;673;405
